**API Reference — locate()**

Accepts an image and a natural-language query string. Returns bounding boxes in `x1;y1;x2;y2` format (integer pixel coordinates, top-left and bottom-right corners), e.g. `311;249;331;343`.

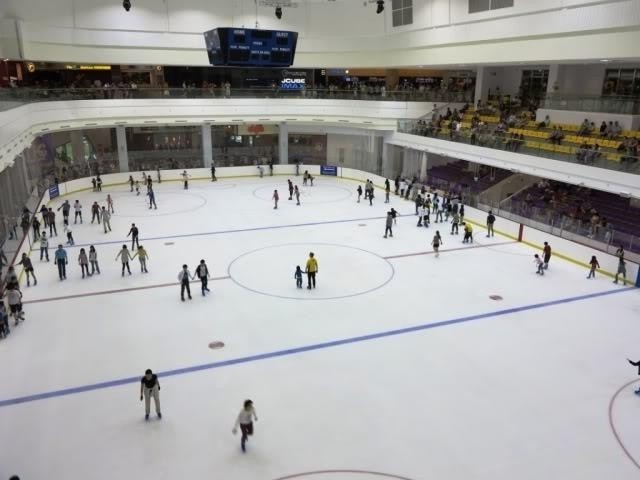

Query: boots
241;443;246;450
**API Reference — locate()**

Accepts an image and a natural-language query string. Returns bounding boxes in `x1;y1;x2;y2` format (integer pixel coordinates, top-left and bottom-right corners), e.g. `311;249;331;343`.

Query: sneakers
357;191;494;257
144;412;161;419
181;296;192;301
9;311;25;326
93;188;102;192
26;211;148;287
587;275;627;286
127;181;162;210
202;289;209;297
273;182;315;211
0;329;10;339
297;285;315;289
536;265;548;275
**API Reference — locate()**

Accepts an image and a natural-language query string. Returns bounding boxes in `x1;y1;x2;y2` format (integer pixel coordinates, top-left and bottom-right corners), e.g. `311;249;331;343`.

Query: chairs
439;102;639;163
427;159;513;196
510;178;639;255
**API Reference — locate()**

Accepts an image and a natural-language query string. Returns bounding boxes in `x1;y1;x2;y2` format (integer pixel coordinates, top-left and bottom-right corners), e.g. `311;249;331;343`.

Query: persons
288;162;314;205
587;245;627;285
232;399;258;452
210;163;216;181
257;162;274;177
164;81;232;98
273;190;279;208
9;475;20;480
513;178;612;243
356;176;495;256
627;358;640;395
417;87;640;172
0;167;161;339
178;259;210;302
533;241;551;274
181;170;191;189
141;369;161;419
293;253;319;291
301;80;465;103
67;80;137;100
